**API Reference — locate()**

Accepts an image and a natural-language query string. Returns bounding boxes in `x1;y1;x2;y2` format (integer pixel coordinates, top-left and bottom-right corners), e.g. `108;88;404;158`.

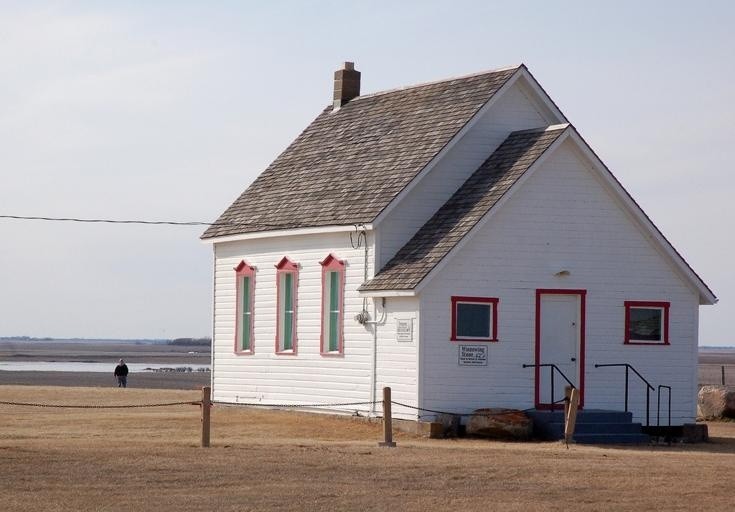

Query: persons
113;359;128;388
